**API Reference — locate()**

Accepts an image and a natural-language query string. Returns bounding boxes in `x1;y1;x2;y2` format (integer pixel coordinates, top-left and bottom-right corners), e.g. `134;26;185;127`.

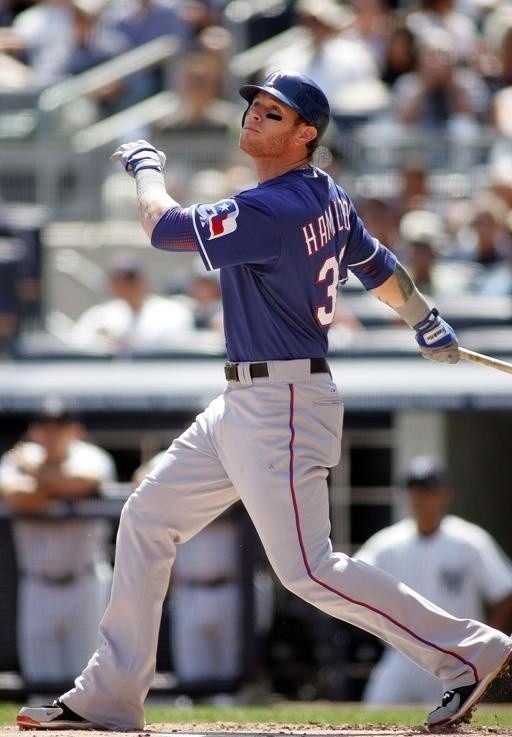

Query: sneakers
428;638;511;733
15;699;101;730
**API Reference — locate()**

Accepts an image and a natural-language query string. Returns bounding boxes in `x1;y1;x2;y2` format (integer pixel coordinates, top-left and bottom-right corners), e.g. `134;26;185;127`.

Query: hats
238;68;331;139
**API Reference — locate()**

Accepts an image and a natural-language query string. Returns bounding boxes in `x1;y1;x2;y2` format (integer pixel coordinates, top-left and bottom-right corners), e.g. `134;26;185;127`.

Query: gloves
109;140;166;176
412;309;459;365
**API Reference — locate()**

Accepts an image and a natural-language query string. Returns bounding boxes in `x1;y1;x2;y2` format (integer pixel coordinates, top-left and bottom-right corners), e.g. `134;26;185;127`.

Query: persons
129;439;253;693
0;396;123;705
14;70;511;734
345;451;512;706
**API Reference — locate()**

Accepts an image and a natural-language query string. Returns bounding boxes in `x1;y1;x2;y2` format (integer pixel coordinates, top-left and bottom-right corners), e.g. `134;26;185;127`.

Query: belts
224;357;330;381
